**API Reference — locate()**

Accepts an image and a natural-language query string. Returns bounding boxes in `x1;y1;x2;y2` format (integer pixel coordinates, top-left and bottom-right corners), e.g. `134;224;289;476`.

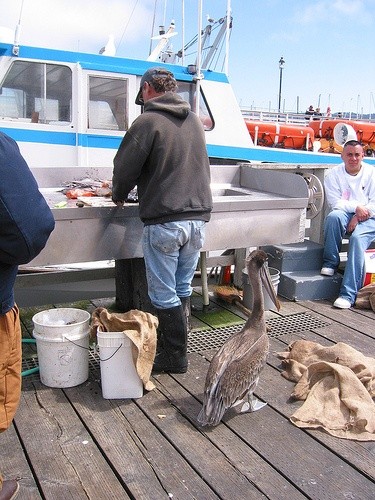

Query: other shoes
0;481;20;500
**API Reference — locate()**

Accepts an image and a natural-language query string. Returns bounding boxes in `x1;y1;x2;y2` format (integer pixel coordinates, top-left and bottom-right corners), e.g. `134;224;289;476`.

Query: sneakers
320;267;335;276
333;297;351;309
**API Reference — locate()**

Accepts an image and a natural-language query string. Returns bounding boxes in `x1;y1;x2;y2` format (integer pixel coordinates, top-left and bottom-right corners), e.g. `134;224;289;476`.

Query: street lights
277;56;286;121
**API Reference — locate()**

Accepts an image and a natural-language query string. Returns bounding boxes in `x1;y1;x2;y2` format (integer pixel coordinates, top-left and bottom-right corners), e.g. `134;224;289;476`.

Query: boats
0;6;374;187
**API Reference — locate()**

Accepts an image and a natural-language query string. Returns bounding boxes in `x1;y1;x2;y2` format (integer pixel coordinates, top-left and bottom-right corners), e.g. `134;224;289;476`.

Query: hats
135;68;174;105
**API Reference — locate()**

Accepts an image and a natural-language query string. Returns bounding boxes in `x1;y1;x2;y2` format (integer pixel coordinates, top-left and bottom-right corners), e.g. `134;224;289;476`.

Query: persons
321;140;375;310
305;104;343;121
0;131;55;500
111;68;213;375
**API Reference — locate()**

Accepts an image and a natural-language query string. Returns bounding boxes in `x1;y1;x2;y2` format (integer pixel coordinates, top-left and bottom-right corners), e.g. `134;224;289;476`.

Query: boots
152;305;187;374
179;296;191;334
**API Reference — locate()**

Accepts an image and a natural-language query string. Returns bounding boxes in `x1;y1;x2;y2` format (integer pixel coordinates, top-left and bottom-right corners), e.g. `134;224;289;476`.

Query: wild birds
197;249;281;428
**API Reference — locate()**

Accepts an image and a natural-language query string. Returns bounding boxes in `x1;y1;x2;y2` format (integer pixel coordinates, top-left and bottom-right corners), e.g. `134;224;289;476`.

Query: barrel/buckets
241;267;280;310
32;308;91;387
97;326;143;400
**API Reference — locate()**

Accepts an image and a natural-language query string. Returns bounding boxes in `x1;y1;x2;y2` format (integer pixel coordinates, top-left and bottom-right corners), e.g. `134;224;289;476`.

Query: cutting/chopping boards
77;196;139;206
